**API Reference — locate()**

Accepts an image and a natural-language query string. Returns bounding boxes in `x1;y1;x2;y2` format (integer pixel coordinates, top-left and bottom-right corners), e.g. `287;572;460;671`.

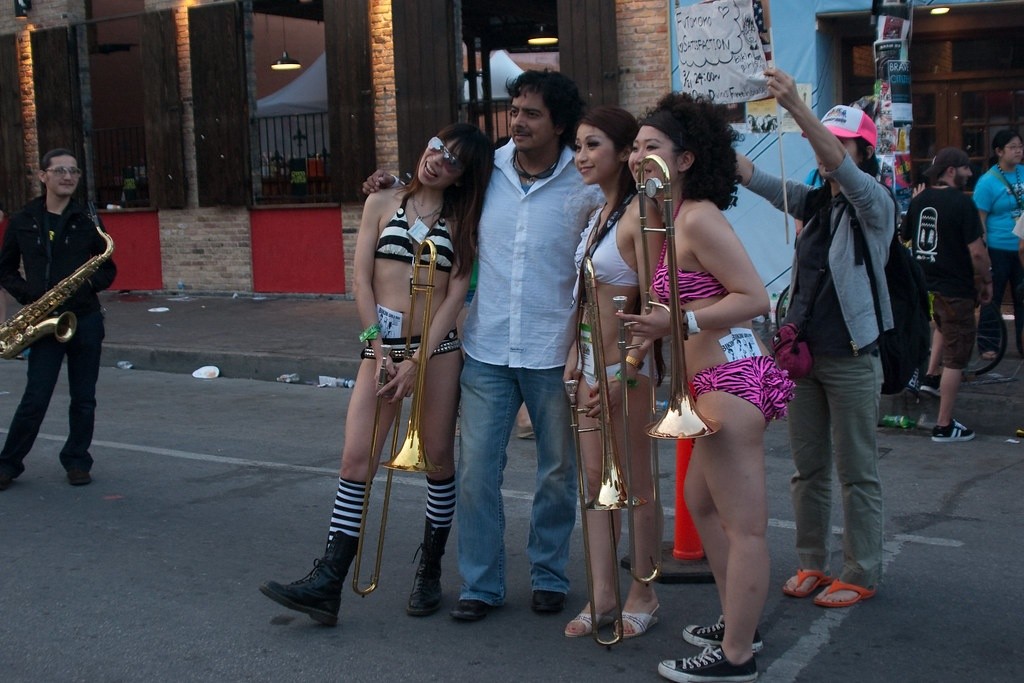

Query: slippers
814;578;876;607
565;612;616;637
613;603;660;637
782;569;832;597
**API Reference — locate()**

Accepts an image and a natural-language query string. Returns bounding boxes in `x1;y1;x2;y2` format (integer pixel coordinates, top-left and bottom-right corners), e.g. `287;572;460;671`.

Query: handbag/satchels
770;321;812;380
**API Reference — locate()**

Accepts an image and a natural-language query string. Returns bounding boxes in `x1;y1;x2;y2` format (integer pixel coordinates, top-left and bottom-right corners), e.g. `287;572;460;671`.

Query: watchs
686;309;701;335
625;355;645;370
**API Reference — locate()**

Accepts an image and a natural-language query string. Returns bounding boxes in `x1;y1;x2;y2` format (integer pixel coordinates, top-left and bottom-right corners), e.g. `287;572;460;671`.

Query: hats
801;104;877;149
921;146;968;182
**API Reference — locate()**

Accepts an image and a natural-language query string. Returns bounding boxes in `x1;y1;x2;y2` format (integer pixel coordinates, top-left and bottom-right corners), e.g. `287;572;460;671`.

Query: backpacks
814;184;932;395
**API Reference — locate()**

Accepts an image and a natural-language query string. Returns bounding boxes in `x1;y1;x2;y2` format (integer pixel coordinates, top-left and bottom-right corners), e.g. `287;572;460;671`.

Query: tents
461;51;530;140
257;51;331;177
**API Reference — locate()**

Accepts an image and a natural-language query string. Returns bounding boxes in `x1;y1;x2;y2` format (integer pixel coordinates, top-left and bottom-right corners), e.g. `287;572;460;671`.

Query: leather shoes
532;589;563;611
449;599;500;619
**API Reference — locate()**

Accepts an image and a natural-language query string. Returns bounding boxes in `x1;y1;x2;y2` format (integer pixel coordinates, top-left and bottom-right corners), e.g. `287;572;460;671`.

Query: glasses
513;150;560;180
44;166;82;178
428;136;466;172
1002;144;1024;151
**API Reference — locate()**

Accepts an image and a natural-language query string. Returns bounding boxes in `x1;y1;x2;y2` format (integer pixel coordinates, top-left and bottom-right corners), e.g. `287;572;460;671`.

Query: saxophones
0;200;114;360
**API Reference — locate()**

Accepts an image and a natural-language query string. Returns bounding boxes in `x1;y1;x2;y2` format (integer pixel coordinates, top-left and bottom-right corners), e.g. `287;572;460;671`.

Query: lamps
270;15;301;70
527;22;558;46
13;0;32;20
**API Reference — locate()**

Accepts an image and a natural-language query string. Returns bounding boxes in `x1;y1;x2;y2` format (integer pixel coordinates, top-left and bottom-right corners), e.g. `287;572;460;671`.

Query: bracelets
358;322;381;342
616;370;640;390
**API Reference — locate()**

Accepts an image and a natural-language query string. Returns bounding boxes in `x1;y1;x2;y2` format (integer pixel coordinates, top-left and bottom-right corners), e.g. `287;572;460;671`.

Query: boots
406;519;452;616
258;531;360;626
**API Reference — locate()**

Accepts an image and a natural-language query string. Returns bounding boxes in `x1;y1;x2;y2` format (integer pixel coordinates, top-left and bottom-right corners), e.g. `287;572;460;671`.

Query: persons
364;68;608;621
0;150;117;491
895;127;1024;442
738;68;897;606
258;118;496;626
569;90;797;683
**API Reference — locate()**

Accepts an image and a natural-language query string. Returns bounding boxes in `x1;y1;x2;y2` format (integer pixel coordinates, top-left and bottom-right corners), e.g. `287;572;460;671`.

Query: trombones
352;237;442;595
567;258;649;648
626;154;722;588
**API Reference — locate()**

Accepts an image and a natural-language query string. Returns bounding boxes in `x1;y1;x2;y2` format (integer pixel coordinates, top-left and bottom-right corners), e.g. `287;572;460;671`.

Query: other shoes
907;368;920;391
983;351;996;359
67;471;91;485
0;475;12;489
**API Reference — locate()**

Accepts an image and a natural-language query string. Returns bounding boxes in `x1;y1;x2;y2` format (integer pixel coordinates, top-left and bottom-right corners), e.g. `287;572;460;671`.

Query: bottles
882;415;916;428
177;278;185;297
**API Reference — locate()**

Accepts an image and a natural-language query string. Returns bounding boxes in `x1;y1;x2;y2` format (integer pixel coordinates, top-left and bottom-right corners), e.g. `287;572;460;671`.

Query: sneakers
919;374;942;396
931;417;975;442
682;615;764;653
658;643;758;682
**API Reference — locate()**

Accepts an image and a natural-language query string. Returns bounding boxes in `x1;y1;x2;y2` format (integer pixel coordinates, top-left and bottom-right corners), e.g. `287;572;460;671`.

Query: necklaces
513;148;562;186
997;162;1022;211
654;197;685;276
412;193;443;226
